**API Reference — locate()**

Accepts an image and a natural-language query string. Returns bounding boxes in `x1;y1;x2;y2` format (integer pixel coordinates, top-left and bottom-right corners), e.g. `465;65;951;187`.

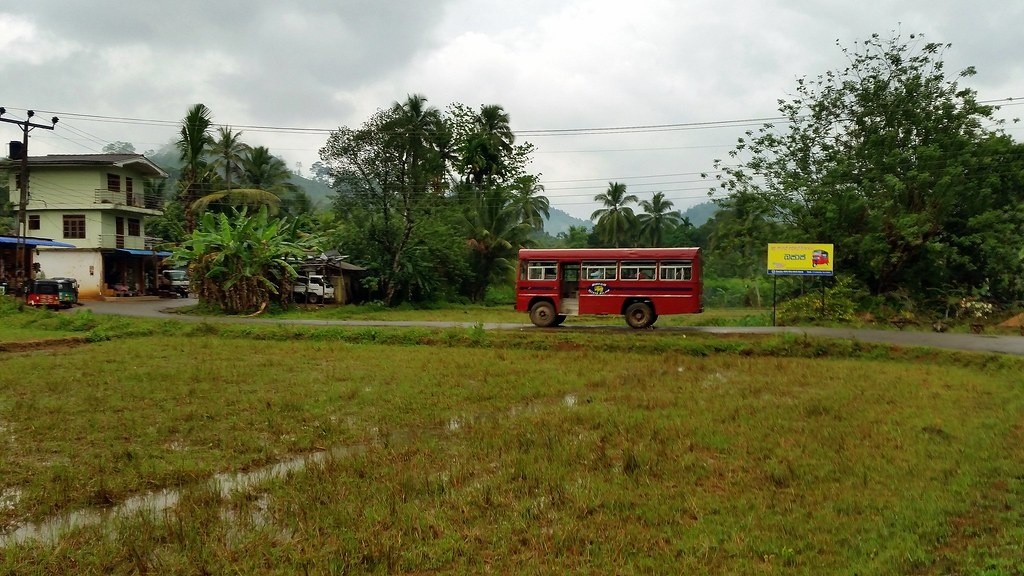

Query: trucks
159;270;189;300
292;274;334;304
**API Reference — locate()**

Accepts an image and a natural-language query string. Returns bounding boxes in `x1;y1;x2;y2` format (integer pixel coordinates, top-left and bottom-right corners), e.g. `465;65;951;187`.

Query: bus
514;247;704;330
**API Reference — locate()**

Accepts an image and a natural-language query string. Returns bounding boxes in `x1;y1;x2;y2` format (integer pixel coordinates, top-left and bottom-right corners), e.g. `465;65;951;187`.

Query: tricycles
50;276;78;310
811;249;829;267
23;278;61;311
